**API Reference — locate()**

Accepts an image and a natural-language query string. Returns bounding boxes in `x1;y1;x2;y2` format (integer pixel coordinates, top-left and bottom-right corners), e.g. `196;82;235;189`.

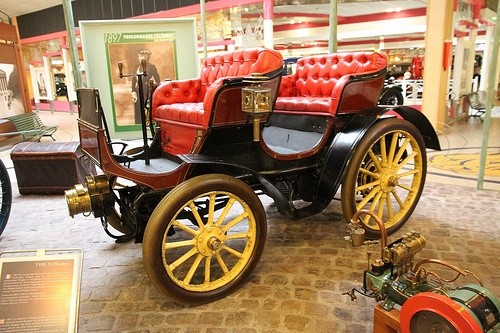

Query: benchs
459;91;494;123
8;112;59;141
149;46;288;166
267;50;389;136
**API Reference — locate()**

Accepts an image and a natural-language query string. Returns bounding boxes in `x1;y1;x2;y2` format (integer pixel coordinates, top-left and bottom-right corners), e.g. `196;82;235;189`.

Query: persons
471;55;484;92
386;70;392;80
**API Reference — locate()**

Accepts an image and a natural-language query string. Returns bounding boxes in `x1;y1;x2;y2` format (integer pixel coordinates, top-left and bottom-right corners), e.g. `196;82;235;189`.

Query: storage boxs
10;141;98;196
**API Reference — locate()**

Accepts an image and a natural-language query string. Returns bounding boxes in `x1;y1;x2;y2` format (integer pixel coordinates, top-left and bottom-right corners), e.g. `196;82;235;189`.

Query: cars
67;47;439;305
